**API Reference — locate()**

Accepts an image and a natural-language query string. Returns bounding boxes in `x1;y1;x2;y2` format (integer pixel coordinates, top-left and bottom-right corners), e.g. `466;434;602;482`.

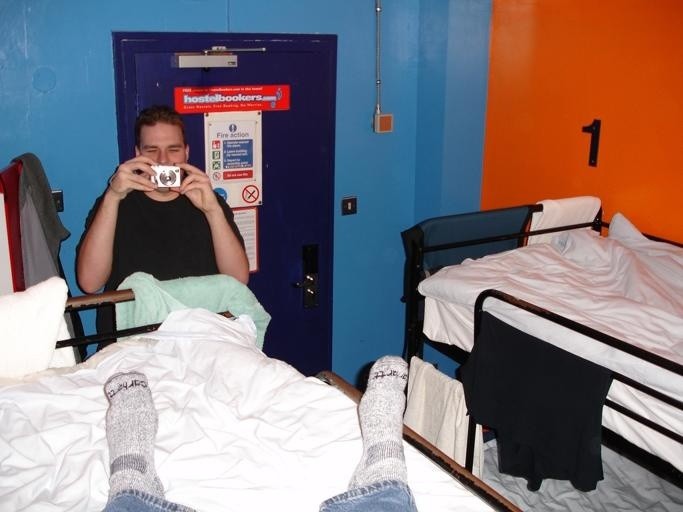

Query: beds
1;274;521;512
403;197;683;489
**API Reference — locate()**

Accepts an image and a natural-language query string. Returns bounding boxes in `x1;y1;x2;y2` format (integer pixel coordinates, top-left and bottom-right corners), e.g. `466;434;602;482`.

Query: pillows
2;276;69;377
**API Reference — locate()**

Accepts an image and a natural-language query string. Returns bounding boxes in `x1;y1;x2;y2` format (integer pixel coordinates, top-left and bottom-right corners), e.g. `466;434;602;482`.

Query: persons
75;106;249;352
104;355;418;512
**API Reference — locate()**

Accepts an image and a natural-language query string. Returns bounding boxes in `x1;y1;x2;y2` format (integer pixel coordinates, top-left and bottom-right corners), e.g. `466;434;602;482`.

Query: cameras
151;165;181;187
